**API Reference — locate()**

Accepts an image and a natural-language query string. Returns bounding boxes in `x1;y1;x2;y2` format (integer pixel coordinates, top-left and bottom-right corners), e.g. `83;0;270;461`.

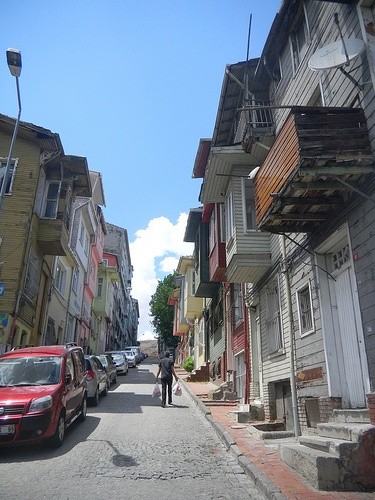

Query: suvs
1;341;89;449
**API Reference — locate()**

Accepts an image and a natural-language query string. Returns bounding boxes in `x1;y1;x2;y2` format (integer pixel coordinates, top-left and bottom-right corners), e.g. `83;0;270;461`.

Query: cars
120;347;148;368
95;354;117;385
106;351;129;376
84;354;110;407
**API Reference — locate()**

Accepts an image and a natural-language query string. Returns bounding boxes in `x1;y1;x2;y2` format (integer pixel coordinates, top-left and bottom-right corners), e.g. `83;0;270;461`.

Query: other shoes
168;402;173;405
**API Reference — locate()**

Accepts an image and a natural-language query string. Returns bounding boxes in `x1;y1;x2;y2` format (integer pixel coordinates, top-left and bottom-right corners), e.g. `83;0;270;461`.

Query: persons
156;351;179;407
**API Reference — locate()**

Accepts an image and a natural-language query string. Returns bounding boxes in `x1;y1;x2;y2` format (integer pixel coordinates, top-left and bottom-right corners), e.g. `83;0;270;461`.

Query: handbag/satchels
172;380;182;396
152;382;161;398
161;404;165;408
